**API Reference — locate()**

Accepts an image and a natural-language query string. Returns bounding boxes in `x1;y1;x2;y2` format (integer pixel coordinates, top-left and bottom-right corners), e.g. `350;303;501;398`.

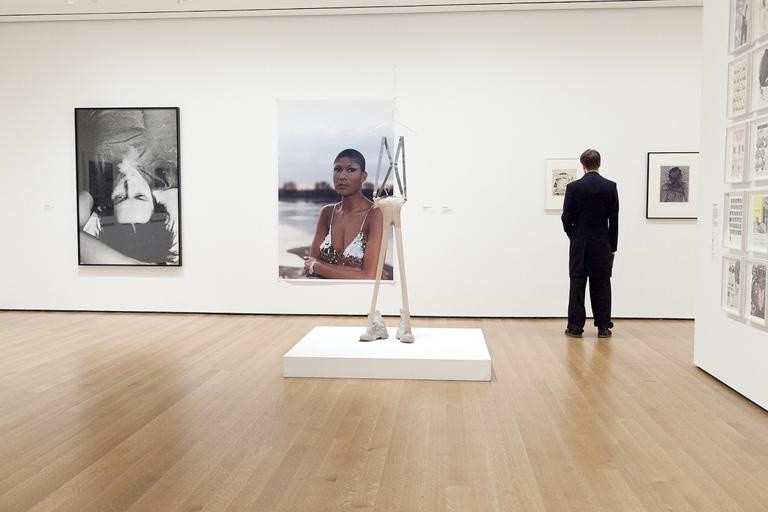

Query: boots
395;308;414;343
358;308;390;341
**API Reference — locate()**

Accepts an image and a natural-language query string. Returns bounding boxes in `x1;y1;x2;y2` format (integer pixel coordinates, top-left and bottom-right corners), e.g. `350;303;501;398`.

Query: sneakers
564;326;583;338
597;326;613;339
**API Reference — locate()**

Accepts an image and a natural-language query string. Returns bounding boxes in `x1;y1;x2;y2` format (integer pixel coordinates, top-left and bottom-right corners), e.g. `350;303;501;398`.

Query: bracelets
307;262;318;275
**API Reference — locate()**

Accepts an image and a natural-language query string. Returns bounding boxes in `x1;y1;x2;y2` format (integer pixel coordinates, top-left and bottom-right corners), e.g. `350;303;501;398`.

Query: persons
559;149;620;338
303;148;392;281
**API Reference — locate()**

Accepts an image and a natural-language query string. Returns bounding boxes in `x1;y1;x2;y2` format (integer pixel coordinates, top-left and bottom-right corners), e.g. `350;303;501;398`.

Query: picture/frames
545;157;585;210
647;152;699;219
720;0;768;329
